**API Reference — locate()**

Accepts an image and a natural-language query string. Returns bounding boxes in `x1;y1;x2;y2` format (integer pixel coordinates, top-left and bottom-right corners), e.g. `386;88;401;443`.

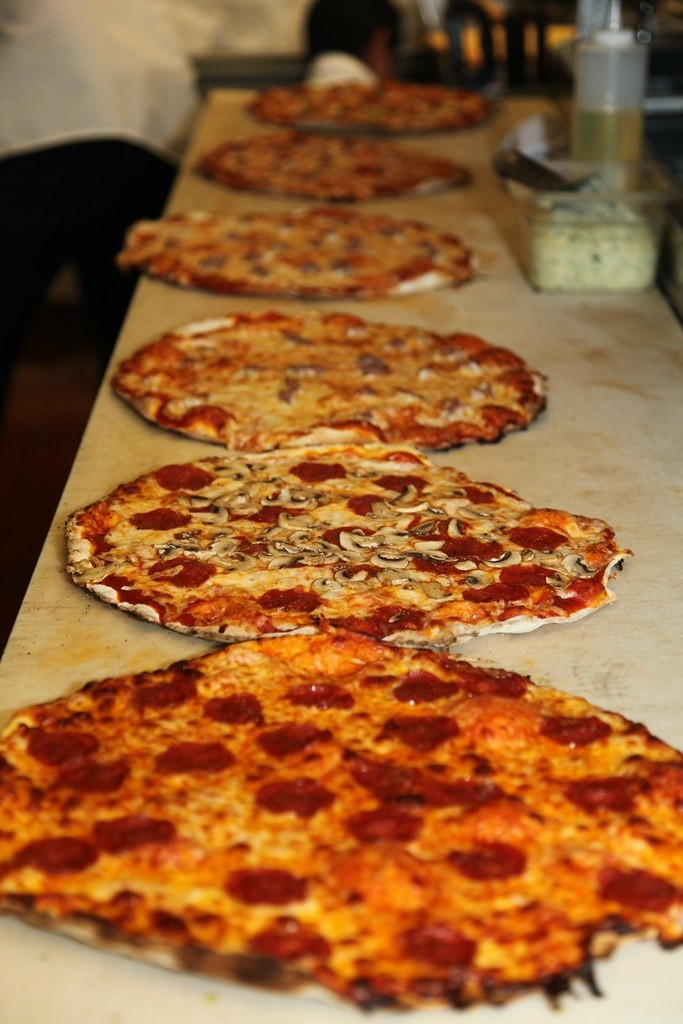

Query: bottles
423;20;543;97
571;0;644;188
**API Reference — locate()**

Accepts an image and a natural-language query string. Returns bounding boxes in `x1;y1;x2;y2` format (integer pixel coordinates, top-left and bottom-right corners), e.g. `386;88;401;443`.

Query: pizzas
0;635;683;1009
62;443;621;639
205;135;457;198
112;312;542;453
256;83;488;129
120;207;472;296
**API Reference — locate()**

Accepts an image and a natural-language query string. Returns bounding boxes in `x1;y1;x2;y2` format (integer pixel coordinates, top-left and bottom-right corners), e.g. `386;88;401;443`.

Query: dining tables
0;87;683;1021
